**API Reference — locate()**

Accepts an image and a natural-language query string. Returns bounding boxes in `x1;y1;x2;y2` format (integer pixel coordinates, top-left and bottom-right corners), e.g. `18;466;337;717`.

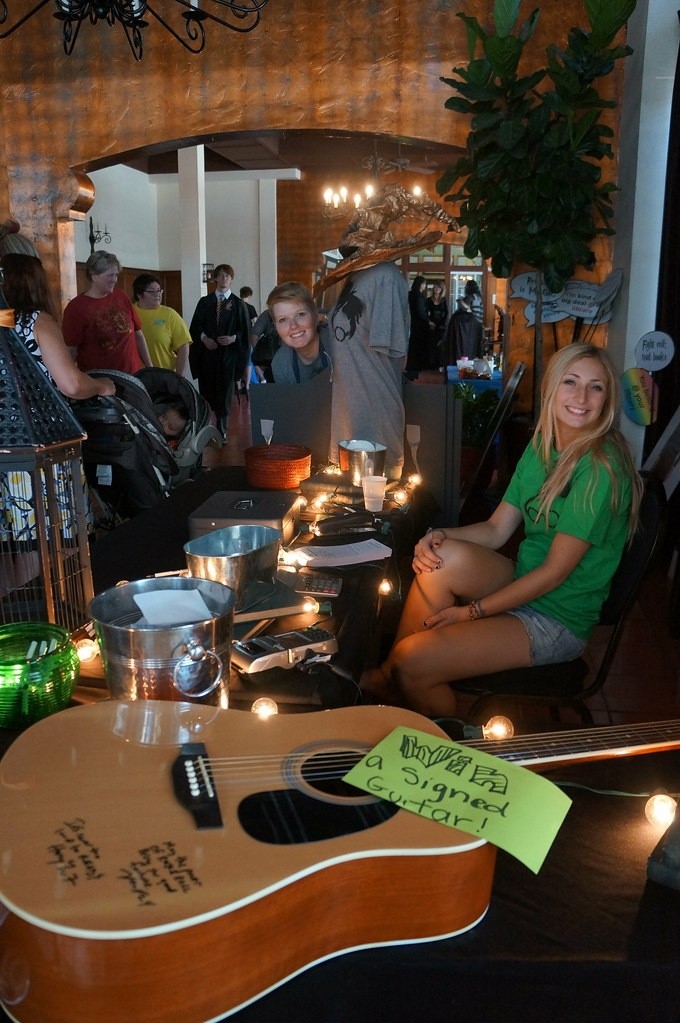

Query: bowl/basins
455;359;474;371
244;444;312;490
0;622;80;733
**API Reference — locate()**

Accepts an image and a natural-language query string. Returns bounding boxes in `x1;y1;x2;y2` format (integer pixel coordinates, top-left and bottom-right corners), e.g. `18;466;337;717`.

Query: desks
0;465;440;715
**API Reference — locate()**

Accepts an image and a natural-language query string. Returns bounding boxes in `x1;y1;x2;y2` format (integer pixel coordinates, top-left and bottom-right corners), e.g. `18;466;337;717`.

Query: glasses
145;289;164;295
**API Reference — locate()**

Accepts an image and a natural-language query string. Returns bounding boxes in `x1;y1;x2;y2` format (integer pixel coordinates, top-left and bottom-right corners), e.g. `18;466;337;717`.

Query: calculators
276;569;343;598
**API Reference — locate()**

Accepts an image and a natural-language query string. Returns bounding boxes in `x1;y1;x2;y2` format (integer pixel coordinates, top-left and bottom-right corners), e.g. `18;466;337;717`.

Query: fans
378;139;439;177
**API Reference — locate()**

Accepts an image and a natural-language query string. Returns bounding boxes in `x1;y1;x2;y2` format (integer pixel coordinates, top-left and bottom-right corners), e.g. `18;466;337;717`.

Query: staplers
312;511;379;545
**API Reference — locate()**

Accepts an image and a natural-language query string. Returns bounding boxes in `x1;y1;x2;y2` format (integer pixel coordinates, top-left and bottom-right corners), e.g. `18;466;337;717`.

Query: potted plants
455;381;520;499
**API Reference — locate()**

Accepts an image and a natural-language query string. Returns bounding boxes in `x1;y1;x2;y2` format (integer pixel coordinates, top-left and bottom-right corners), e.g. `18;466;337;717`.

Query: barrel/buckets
183;524;283;613
336;439;387;487
88;577;238;709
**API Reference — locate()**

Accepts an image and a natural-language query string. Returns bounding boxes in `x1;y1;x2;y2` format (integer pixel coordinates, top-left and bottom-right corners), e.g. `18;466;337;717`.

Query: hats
456;296;473;311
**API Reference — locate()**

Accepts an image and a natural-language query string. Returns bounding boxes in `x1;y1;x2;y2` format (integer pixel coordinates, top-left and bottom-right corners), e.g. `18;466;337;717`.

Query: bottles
485;356;494;375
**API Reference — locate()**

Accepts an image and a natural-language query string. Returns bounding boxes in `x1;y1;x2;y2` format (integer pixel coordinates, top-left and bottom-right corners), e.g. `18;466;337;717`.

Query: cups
361;475;387;512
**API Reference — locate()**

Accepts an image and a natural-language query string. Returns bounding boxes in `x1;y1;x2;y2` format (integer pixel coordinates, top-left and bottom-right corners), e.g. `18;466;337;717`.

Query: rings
413;555;417;561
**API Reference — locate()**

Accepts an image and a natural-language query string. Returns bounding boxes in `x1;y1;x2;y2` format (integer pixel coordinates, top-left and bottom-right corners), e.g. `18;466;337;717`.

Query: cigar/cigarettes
13;639;62;716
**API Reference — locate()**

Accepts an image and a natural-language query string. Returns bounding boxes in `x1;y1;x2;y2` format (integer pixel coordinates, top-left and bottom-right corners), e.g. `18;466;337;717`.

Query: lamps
202;263;217;284
321;136;421;221
0;0;269;61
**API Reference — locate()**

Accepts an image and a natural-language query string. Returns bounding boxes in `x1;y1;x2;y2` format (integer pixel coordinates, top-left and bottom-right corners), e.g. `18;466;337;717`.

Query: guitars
1;703;680;1023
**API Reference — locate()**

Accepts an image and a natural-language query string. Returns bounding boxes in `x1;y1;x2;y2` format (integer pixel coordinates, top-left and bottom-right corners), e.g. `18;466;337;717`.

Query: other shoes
221;433;229;443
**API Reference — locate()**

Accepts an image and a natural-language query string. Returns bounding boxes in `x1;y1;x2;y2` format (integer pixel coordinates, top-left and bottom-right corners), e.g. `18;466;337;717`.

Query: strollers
69;366;222;518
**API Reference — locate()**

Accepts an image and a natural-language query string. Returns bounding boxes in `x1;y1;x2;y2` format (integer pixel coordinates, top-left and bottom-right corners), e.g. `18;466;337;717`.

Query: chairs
447;469;668;728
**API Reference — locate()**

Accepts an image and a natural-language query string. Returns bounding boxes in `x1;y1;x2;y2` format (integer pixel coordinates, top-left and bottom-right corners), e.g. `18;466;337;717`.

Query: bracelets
426;527;441;534
468;600;483;621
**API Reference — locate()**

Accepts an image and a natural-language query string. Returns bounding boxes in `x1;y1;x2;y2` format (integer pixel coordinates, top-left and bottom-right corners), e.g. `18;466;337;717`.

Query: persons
267;281;332;384
0;253;116;541
236;286;258;392
189;264;252;443
62;250;155;375
328;184;463;480
132;273;193;378
246;309;274;390
408;276;483;368
364;343;644;718
158;405;188;450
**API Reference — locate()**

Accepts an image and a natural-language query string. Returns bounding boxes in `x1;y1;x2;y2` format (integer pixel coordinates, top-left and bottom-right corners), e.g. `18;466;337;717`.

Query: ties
216;295;223;324
